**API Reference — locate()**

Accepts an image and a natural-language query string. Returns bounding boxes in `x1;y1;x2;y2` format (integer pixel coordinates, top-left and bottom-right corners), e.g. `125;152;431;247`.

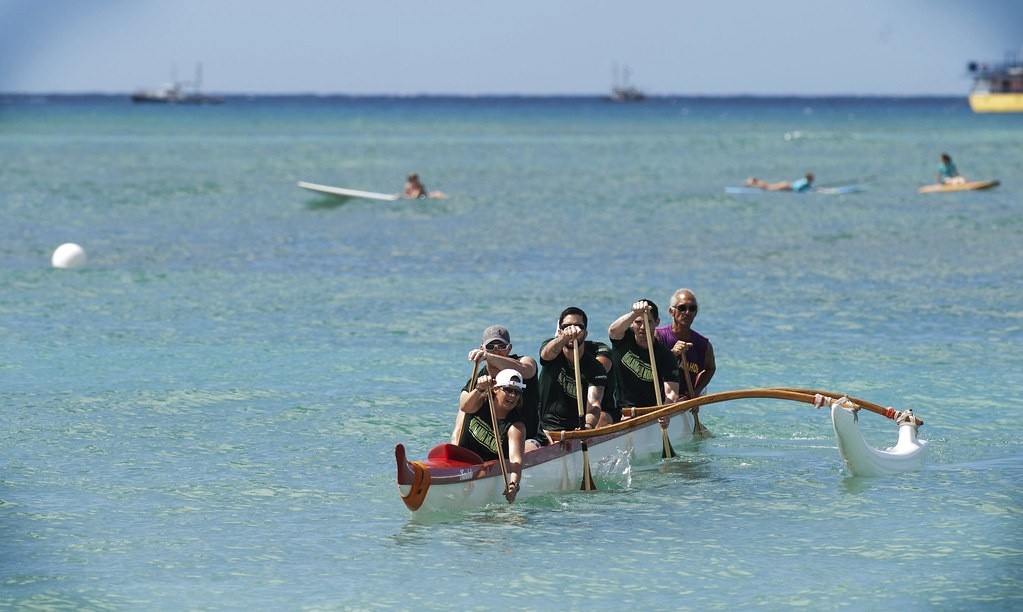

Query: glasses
560;322;585;331
500;387;523;397
486;344;509;351
671;304;697;312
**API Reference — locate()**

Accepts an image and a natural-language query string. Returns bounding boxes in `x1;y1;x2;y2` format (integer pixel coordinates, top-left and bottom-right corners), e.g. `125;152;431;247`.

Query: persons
608;300;679;408
555;320;622;428
655;288;716;401
458;369;526;503
535;307;607;446
404;172;446;199
746;172;813;192
936;154;958;184
468;325;539;440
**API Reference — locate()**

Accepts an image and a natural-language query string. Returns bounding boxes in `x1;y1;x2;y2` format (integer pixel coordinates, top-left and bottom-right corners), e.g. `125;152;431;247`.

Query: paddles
572;338;597;490
485;379;513;506
681;349;708;435
643;310;677;458
456;355;486;447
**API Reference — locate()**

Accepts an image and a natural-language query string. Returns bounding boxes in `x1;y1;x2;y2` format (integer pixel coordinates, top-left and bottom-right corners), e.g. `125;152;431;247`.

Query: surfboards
297;179;397;201
918;178;1001;193
722;185;869;195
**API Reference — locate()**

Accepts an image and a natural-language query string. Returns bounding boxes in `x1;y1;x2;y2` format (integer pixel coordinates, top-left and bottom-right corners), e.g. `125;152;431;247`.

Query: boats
130;91;224;107
613;83;648;104
968;87;1023;114
296;179;449;208
395;389;927;517
955;48;1023;93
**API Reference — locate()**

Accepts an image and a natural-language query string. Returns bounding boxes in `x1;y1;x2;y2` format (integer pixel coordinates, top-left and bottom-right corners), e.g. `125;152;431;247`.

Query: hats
482;325;510;347
489;369;526;390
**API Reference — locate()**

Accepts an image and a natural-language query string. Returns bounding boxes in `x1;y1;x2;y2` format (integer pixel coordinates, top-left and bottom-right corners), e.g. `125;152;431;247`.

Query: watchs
510;483;520;491
